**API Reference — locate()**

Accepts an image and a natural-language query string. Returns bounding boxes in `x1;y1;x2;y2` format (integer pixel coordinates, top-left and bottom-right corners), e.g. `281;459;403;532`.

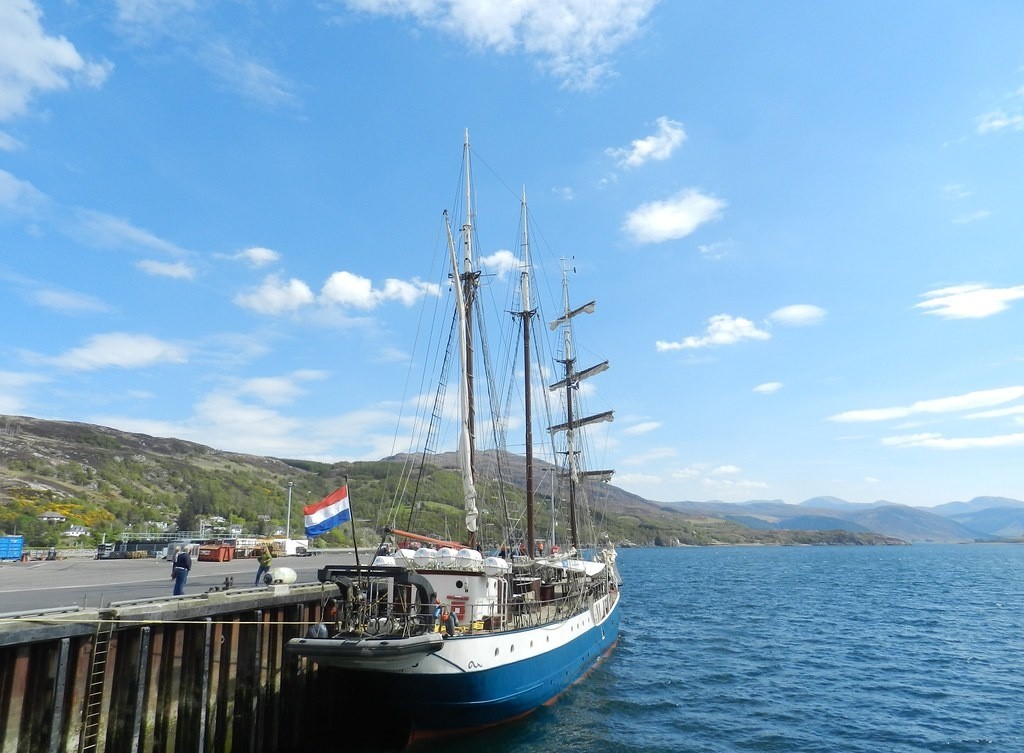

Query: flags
304;484;352;540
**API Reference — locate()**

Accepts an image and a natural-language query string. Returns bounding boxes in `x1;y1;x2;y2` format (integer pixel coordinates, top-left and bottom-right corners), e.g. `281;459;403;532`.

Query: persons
255;548;272;587
171;546;192;600
477;541;560;559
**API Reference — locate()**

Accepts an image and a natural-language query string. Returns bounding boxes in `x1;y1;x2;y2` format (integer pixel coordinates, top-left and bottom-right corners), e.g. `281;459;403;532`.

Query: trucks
296;542;322;557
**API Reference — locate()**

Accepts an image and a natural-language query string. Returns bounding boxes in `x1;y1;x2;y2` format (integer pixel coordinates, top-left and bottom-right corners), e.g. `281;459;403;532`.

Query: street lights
309;537;313;547
284;482;293;557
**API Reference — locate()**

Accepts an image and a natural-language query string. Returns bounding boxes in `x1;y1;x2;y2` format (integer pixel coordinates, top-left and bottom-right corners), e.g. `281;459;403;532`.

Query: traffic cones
21;554;26;562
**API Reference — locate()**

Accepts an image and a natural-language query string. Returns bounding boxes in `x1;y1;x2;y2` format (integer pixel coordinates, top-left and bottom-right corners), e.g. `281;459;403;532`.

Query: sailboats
286;126;623;740
